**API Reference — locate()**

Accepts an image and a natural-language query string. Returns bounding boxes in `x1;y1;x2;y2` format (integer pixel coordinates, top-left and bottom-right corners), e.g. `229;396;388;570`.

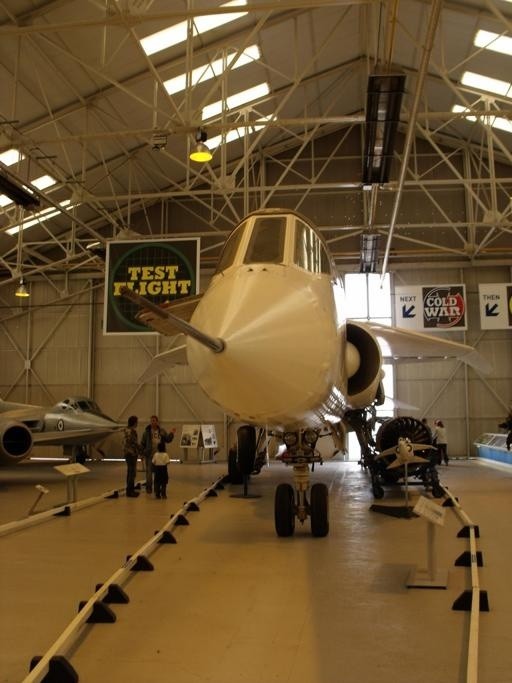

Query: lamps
14;276;29;297
189;127;212;162
363;71;408;184
360;233;380;273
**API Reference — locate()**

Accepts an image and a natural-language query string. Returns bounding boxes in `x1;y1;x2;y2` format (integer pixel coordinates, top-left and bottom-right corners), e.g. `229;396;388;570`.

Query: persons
121;415;139;497
138;415;176;494
421;418;431;435
152;442;170;498
432;419;449;466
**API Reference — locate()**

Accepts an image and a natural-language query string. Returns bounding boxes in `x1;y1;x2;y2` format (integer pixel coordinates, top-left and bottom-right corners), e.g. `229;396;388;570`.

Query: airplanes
116;202;491;541
1;395;129;470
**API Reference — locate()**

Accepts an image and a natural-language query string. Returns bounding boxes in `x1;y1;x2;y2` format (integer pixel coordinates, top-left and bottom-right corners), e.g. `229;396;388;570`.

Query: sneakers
126;490;140;497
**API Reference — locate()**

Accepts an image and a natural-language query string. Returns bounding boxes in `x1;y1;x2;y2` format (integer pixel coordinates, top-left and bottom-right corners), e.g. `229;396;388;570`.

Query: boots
145;485;167;498
